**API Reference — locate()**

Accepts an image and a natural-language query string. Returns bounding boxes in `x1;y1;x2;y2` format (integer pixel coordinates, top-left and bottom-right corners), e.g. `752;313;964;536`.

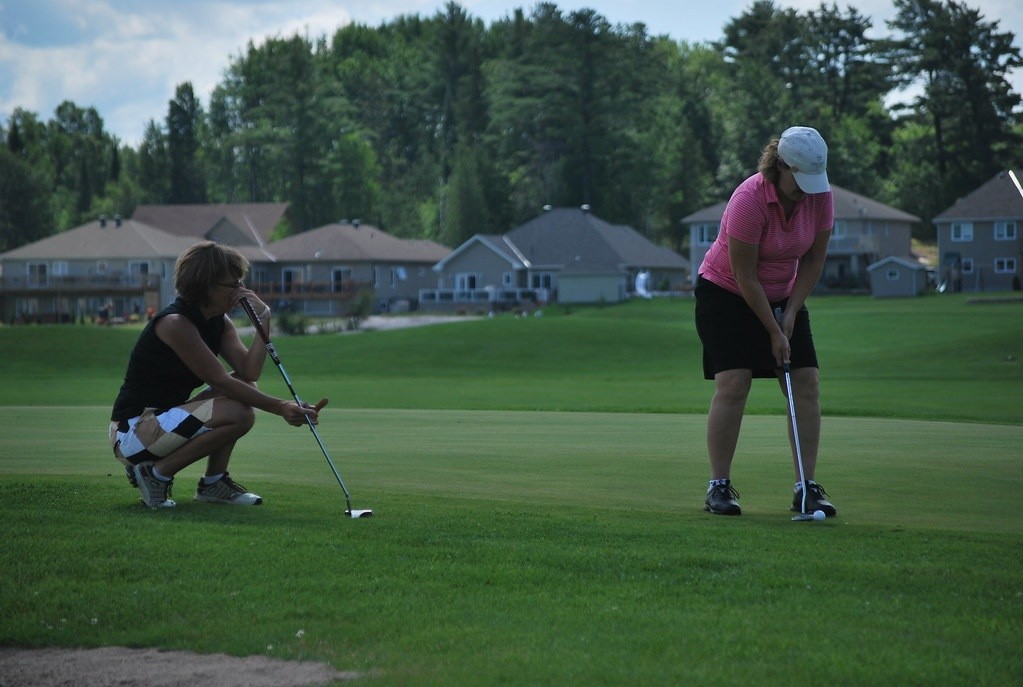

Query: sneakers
790;480;836;516
126;461;176;508
194;471;262;505
704;480;741;515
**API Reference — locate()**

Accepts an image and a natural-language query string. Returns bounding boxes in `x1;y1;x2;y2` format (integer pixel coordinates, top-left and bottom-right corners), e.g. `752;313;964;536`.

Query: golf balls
813;510;826;521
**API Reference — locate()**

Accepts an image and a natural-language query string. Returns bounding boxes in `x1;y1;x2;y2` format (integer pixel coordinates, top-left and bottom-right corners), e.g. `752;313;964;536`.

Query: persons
693;126;838;517
109;243;318;507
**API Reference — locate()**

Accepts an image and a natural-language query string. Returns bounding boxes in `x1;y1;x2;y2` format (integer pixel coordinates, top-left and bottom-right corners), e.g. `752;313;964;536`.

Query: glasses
215;280;239;289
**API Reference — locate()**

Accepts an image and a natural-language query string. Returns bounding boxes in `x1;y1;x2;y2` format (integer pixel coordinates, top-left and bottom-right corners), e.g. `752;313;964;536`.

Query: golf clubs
239;297;374;517
774;306;814;521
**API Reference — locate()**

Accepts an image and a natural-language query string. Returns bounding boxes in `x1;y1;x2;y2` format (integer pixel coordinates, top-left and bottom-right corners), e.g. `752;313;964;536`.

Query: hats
777;127;831;194
